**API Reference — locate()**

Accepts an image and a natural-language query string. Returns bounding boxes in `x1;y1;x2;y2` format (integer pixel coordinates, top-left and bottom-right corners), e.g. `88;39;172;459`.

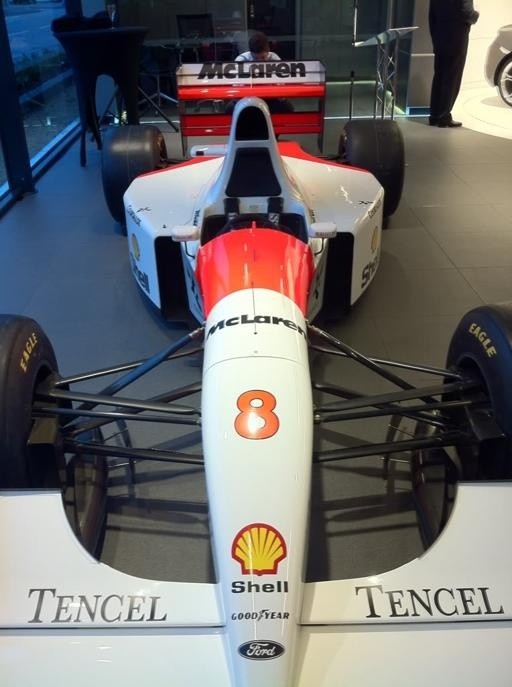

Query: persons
224;34;295;114
429;0;480;128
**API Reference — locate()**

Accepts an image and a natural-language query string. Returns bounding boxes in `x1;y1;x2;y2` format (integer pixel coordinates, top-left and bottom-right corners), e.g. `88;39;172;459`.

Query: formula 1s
1;61;512;686
